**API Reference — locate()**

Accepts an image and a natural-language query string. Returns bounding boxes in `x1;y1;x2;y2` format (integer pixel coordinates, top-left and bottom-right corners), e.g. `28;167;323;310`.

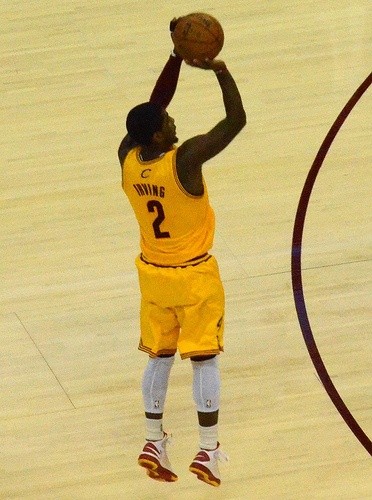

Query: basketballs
172;11;226;67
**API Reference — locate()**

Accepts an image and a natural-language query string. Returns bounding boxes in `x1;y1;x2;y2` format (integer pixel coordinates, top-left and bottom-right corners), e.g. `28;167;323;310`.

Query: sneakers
187;441;230;488
137;432;180;482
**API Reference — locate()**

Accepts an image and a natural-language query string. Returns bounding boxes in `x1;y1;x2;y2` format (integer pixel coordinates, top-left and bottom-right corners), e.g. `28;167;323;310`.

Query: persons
119;14;247;487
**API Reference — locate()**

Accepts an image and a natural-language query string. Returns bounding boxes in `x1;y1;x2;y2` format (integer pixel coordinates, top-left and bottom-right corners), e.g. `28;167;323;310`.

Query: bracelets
215;64;227;75
